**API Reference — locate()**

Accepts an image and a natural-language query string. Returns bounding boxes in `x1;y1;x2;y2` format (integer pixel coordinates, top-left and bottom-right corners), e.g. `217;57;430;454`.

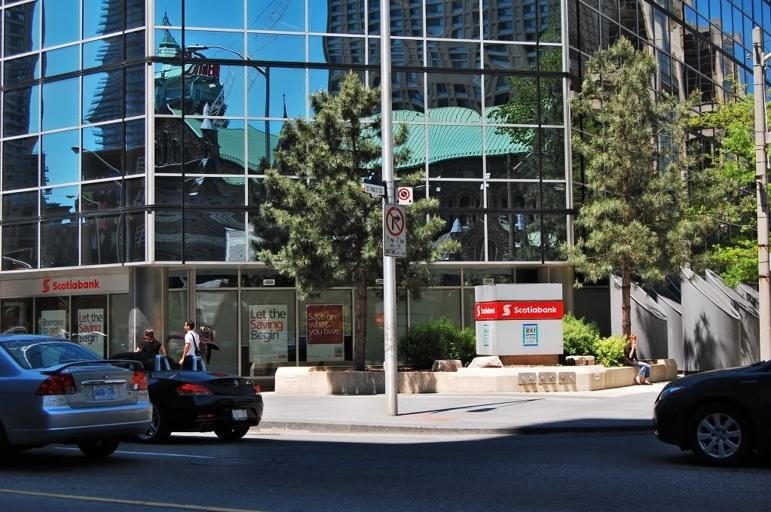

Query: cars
654;359;771;466
0;335;263;459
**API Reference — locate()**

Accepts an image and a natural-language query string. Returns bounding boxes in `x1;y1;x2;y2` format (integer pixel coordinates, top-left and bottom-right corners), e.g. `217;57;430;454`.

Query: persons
625;335;654;384
179;319;200;370
137;328;167;372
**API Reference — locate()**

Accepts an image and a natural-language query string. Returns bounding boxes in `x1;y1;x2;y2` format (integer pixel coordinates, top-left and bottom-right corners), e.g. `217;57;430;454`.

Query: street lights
66;146;130;264
187;43;271;175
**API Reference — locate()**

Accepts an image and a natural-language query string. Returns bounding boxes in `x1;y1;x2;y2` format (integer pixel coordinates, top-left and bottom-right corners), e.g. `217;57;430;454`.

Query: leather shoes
634;376;652;385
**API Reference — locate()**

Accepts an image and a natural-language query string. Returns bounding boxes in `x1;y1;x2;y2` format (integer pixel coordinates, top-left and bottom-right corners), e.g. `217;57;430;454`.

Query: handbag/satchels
188;332;203;356
